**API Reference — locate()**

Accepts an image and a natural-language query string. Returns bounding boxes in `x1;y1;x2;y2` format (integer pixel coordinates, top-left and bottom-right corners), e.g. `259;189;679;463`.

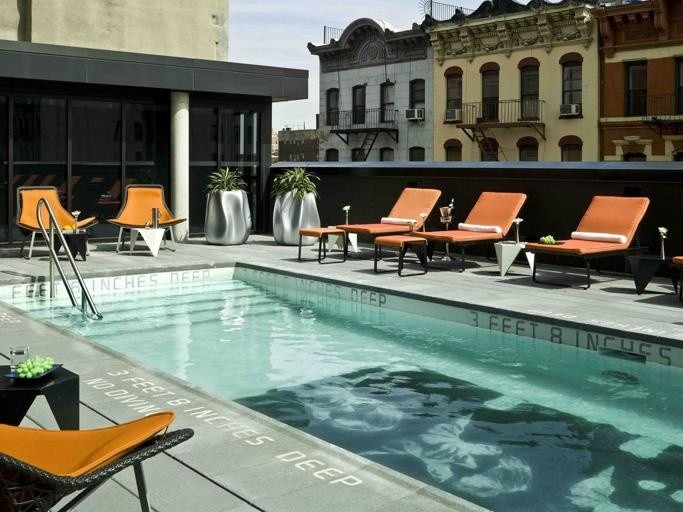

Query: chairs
0;409;194;510
10;184;187;261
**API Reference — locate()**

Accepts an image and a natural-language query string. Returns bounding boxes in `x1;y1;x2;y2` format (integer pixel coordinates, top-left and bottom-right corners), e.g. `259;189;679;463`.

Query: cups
9;343;30;372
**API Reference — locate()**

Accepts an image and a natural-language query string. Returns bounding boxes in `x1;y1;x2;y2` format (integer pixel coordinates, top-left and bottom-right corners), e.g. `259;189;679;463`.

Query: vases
659;240;665;260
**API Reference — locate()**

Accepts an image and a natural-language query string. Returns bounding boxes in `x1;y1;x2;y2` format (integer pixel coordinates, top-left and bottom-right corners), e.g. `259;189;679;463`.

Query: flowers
657;227;668;240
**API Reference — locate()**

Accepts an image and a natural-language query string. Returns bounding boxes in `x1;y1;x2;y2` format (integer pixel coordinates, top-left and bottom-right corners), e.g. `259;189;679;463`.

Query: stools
298;227;347;265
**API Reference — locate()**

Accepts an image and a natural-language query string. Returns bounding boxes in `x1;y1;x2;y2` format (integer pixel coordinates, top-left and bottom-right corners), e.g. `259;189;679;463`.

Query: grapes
16;354;55;378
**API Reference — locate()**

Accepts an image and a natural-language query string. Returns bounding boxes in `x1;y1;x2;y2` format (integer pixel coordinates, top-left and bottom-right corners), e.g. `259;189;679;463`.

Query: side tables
0;363;80;430
626;254;678;295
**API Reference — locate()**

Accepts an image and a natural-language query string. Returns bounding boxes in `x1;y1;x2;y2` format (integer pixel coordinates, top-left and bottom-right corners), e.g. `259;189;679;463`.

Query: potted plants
198;164;323;247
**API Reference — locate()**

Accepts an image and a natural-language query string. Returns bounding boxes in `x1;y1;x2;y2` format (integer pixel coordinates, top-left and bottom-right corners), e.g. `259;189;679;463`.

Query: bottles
151;207;159;227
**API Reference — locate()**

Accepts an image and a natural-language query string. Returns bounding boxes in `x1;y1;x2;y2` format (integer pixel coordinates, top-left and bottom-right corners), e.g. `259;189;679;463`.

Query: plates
3;363;64;381
